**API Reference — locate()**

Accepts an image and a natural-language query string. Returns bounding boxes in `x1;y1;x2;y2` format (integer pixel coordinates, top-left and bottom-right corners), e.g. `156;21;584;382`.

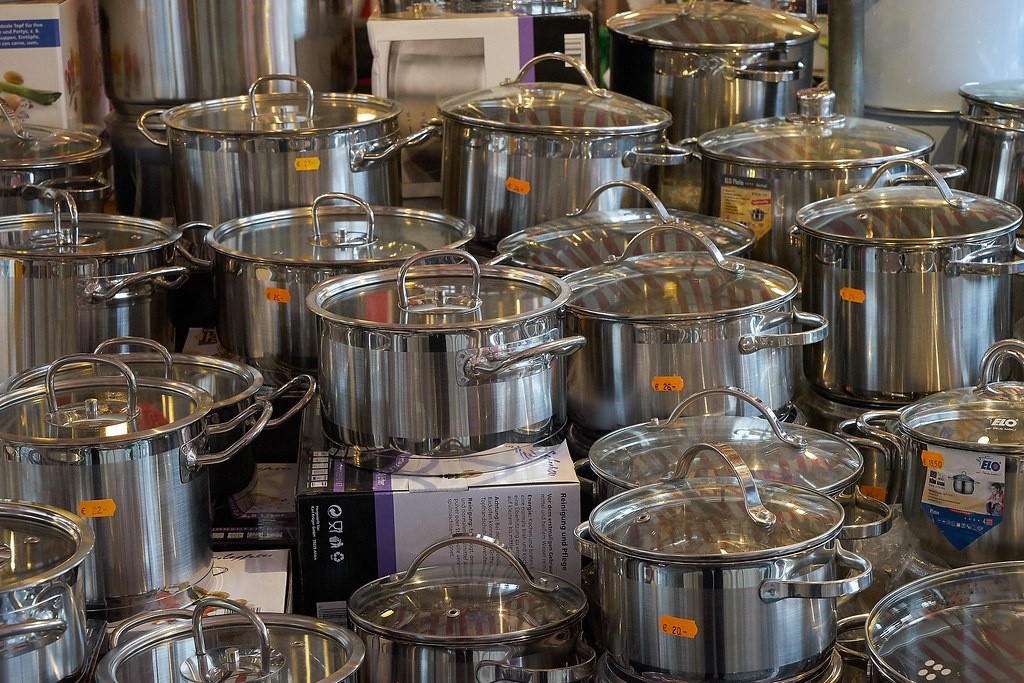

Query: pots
0;0;1024;683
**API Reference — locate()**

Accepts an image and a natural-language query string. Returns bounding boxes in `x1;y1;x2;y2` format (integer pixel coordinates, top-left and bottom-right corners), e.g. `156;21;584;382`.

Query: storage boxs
367;6;596;199
86;519;292;656
208;465;304;616
0;0;117;136
182;327;304;462
293;393;582;627
